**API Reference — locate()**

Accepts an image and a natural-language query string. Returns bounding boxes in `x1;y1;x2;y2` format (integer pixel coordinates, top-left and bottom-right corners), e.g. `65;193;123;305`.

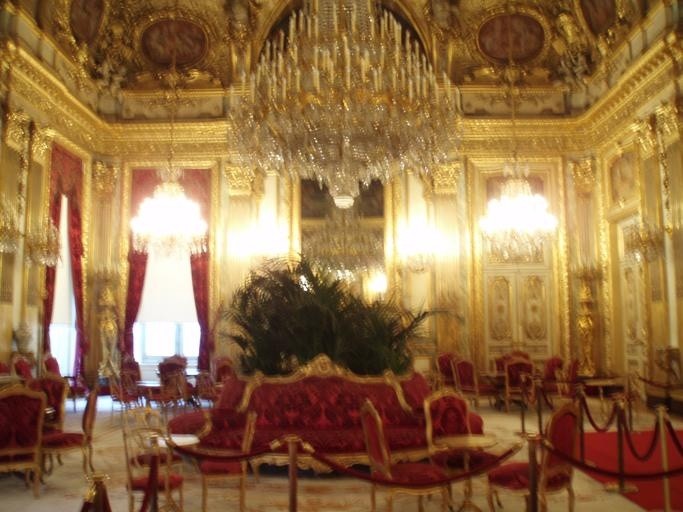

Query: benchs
236;353;449;484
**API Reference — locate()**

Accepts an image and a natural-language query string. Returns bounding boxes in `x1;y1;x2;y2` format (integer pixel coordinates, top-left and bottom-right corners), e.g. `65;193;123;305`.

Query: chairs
354;347;589;512
0;346;260;511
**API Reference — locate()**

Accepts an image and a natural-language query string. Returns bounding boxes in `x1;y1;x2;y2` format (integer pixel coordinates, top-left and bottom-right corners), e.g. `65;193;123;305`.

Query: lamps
221;0;469;214
